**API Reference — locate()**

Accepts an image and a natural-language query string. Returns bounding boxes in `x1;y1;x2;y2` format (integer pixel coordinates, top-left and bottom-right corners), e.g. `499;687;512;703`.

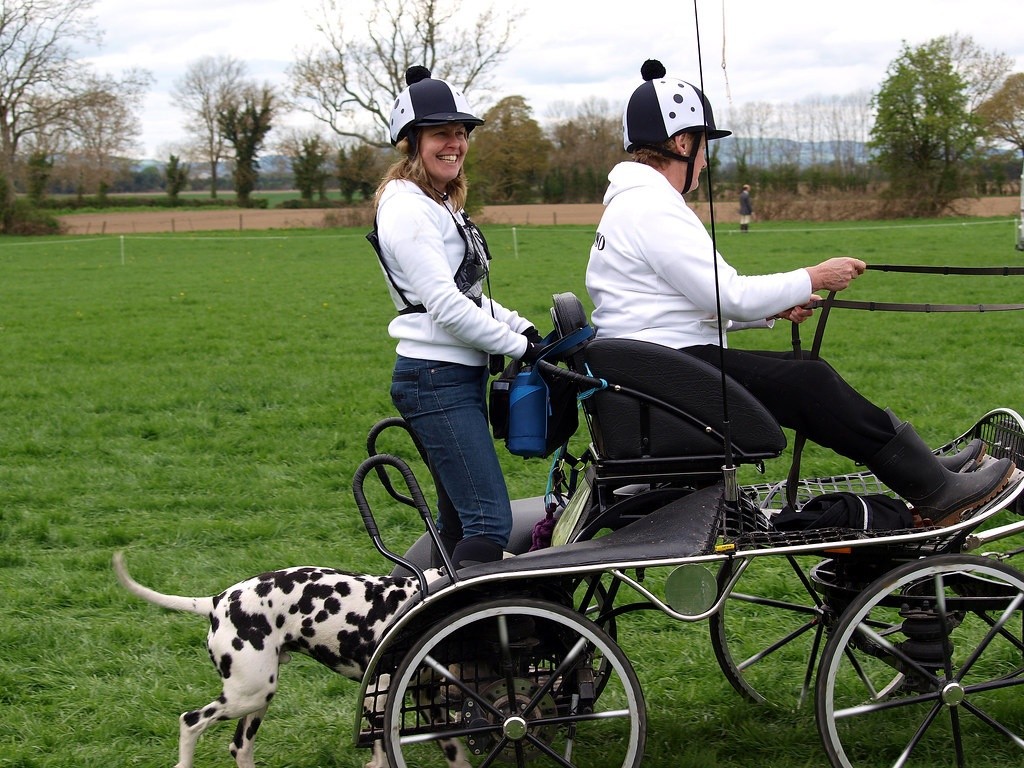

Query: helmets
389;64;486;146
622;57;732;153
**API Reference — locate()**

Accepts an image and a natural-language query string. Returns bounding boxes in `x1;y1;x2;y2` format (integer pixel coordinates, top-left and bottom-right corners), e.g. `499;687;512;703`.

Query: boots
390;529;504;580
865;406;1016;530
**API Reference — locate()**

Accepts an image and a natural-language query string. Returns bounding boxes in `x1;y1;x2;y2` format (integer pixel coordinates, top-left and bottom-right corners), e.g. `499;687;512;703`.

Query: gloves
522;325;560;368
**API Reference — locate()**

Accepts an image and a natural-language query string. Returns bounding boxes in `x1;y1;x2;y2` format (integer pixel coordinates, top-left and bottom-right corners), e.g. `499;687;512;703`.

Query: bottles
508;364;547;454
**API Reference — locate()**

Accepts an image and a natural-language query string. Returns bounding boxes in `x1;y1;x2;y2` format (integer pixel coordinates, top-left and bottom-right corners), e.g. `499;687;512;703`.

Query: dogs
110;551;470;767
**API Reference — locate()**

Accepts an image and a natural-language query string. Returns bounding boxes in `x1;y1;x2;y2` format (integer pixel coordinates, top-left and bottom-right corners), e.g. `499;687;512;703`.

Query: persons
368;64;542;710
586;60;1014;527
737;185;753;231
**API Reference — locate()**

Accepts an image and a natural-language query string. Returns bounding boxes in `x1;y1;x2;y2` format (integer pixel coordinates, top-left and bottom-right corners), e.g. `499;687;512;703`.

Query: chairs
548;292;790;469
